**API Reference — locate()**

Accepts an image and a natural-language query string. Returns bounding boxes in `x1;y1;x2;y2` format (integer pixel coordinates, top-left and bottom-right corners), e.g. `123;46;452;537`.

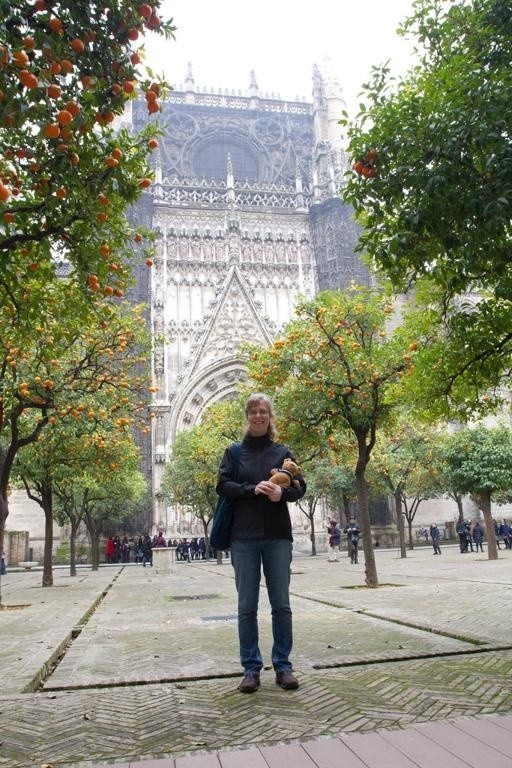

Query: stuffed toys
270;456;303;489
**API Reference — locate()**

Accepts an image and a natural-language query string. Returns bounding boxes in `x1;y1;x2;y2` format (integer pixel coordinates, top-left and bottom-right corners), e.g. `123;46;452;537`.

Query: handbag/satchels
351;534;359;542
210;494;233;550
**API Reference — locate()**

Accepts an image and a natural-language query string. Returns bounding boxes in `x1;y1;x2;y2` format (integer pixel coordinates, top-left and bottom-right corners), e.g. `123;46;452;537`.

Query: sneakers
240;673;260;692
275;670;299;689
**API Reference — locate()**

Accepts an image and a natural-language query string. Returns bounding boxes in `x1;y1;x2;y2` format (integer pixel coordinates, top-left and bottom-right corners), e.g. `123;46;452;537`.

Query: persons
492;517;511;549
454;515;485;552
425;525;448;554
327;519;342;561
345;518;362;563
104;531;228;564
216;392;306;692
